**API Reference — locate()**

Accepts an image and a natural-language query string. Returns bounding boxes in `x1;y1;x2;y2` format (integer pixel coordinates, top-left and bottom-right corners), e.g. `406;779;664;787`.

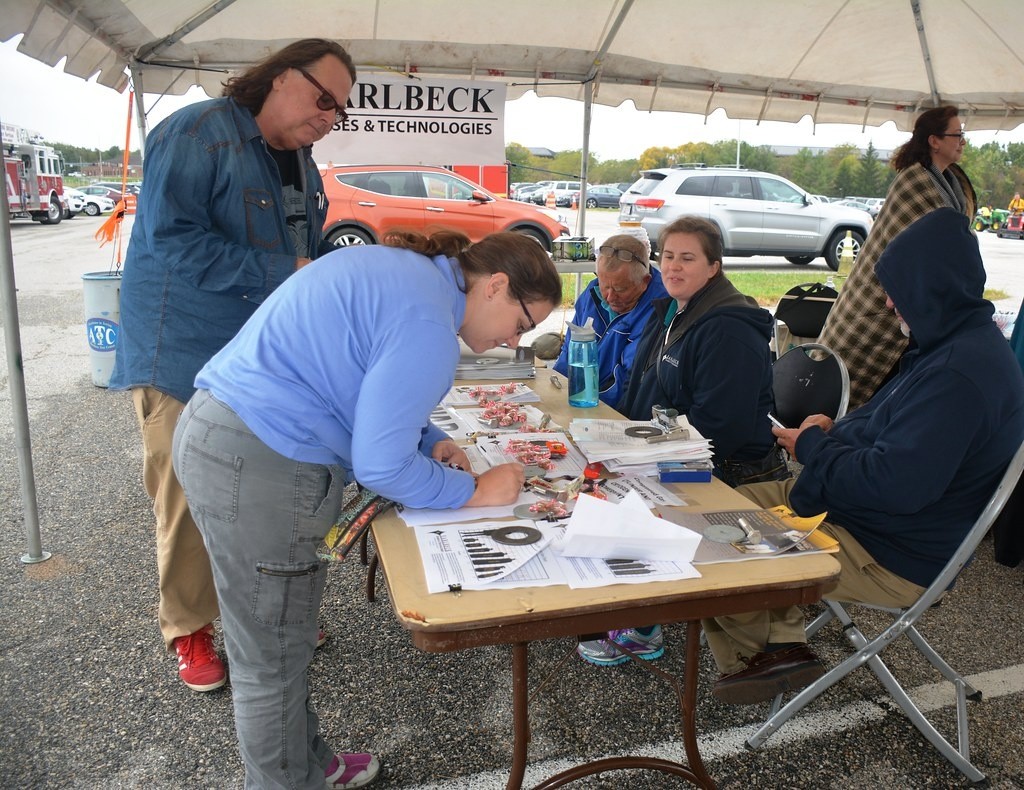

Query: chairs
769;282;841;359
743;443;1024;782
768;343;850;468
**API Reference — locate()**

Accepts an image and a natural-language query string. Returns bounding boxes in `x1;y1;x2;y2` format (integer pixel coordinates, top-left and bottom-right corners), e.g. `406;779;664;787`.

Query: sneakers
316;630;326;647
325;754;379;789
173;622;226;691
576;624;664;666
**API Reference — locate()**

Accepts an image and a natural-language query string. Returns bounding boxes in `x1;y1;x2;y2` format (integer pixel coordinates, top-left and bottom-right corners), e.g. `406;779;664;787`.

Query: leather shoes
713;646;825;703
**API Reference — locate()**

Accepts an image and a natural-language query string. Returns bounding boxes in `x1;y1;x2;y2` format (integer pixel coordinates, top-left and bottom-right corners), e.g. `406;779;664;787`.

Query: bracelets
473;476;478;491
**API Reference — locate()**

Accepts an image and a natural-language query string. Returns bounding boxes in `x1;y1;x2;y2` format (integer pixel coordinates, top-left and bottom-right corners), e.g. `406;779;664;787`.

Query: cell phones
768;410;789;431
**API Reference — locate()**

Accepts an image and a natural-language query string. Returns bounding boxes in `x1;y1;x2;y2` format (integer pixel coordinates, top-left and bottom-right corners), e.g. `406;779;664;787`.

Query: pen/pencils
474;440;496;468
466;432;518;436
442;462;459;469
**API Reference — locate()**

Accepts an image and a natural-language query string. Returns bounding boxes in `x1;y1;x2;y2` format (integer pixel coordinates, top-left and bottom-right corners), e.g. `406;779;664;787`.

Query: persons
702;205;1024;702
1008;191;1024;214
801;107;978;411
547;217;791;668
110;39;356;693
169;231;563;790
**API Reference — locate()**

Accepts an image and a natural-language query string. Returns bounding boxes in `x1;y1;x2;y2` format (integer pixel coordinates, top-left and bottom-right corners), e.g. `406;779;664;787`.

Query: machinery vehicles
997;207;1024;239
971;207;1010;233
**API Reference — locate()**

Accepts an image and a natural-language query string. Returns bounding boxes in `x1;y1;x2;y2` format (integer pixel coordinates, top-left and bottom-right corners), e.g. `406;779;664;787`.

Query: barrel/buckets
80;270;124;388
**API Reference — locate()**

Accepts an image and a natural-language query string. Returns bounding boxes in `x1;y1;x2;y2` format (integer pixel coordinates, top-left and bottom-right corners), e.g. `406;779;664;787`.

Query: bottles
824;276;836;291
565;317;600;408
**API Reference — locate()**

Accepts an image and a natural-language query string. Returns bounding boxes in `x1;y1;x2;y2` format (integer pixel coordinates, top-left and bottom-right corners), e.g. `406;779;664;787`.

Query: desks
356;340;839;790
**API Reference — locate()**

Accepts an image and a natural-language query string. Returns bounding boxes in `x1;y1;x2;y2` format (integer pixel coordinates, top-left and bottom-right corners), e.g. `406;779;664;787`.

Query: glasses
933;131;966;142
291;66;348;123
509;288;536;335
598;245;650;269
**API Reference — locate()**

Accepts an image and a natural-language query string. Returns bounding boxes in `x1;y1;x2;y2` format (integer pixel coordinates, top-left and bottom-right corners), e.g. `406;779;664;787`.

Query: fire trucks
1;124;65;225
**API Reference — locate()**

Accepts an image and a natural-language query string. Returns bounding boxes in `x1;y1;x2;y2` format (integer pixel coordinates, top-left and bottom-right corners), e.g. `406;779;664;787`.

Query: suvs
618;164;875;271
95;183;141;197
316;166;570;253
612;183;632;193
543;181;593;205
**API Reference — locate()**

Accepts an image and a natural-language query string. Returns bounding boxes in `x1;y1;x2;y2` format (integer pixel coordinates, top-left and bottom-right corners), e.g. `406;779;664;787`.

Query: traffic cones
836;231;854;276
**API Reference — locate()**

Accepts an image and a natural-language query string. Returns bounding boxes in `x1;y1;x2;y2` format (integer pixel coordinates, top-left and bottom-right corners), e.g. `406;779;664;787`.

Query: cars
509;181;551;204
573;185;624;209
74;187;123;205
62;186;116;216
58;190;87;218
812;195;885;221
